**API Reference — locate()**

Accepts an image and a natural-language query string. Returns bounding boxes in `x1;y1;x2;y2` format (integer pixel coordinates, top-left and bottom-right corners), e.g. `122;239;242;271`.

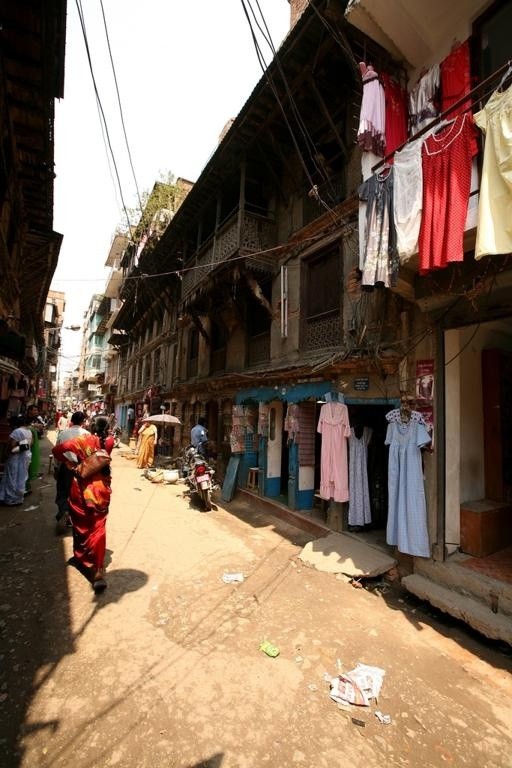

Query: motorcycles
112;426;123;448
165;430;221;511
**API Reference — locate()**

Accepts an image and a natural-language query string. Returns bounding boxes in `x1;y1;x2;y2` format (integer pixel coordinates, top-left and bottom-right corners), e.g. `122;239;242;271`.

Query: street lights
46;324;80;331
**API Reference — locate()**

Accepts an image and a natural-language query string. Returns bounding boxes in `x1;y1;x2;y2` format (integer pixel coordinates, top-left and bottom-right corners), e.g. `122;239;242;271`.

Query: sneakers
92;577;108;595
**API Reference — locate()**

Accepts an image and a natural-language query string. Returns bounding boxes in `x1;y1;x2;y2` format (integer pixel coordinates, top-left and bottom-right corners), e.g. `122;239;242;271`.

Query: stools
246;467;259;489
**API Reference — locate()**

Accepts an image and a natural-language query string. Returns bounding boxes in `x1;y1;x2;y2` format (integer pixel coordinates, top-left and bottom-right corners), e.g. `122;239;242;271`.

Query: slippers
6;472;44;506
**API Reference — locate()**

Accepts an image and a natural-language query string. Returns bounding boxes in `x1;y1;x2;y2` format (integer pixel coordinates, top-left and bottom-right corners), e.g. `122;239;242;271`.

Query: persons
191;418;208;457
126;405;135;433
0;403;41;506
54;398;115;592
137;419;158;470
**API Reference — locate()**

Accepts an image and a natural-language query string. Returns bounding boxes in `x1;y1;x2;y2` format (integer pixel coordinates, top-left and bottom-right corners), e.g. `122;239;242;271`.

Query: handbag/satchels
11;428;30;454
73;449;113;479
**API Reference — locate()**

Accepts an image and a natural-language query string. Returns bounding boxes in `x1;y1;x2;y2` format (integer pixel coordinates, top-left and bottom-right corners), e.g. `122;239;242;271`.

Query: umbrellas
144;411;183;444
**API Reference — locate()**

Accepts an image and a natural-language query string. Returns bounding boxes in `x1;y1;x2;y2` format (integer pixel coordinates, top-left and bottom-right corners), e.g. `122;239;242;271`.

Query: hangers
387;390;424;429
361;35;512;182
323;391;346;409
